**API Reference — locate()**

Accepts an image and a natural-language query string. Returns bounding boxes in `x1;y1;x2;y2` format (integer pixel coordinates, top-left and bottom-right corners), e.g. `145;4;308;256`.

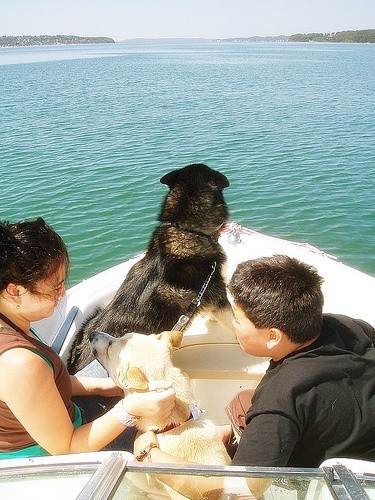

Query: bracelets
135;442;160;461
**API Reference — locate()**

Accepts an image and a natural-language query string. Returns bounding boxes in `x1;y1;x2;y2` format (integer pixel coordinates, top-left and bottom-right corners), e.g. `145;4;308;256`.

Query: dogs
89;331;234;500
68;163;234;381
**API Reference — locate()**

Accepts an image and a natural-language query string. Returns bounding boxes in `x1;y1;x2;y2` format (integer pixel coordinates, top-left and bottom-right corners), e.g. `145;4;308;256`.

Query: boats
0;220;375;499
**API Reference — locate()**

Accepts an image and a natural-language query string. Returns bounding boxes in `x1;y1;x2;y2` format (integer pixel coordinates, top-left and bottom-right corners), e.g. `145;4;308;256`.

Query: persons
0;216;178;462
133;253;375;500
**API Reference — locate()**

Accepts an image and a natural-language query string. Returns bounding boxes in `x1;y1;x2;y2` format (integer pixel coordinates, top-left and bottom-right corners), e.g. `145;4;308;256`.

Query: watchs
114;399;141;428
